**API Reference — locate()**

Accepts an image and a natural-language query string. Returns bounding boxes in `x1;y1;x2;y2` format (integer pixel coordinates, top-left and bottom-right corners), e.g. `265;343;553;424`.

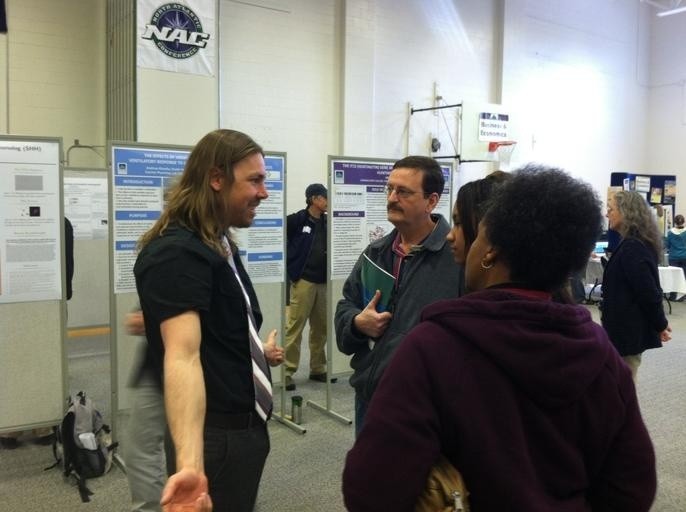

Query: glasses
383;186;426;197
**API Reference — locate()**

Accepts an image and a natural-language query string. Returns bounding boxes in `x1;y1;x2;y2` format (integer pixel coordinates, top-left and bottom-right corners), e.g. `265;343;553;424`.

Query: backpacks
56;392;114;477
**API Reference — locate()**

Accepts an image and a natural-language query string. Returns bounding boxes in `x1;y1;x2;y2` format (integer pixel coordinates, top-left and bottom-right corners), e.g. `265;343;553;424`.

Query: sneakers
284;377;296;391
310;372;338;383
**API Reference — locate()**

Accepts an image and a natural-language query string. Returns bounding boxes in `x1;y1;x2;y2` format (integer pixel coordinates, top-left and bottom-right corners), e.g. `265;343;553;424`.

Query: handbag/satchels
414;453;471;512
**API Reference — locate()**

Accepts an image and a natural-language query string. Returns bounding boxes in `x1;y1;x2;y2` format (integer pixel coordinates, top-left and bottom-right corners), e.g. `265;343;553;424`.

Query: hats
306;184;328;197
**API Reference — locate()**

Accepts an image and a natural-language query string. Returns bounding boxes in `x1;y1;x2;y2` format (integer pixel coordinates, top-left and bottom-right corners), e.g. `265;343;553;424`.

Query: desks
586;258;686;315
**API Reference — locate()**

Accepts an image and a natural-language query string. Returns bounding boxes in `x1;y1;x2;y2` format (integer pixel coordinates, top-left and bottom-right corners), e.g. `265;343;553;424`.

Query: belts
205;406;273;430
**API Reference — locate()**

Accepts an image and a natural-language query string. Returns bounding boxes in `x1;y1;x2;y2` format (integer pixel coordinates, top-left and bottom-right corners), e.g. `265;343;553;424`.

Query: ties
221;234;274;423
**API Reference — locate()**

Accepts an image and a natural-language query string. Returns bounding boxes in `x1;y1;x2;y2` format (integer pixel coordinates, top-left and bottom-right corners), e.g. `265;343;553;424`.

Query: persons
120;294;173;512
343;167;658;509
598;189;674;382
662;215;686;302
276;183;339;391
445;170;523;292
333;154;464;442
132;128;288;512
